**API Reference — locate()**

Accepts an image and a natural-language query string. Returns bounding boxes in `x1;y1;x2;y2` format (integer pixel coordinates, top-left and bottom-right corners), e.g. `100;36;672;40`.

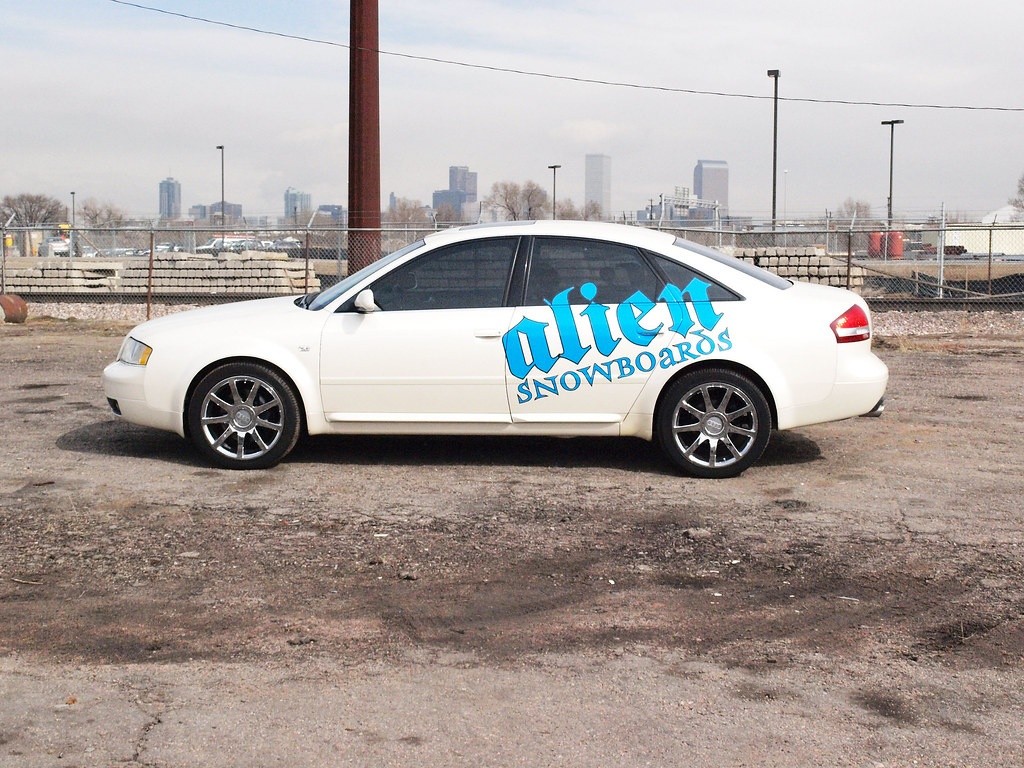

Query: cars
82;237;302;257
38;236;70;257
102;216;888;480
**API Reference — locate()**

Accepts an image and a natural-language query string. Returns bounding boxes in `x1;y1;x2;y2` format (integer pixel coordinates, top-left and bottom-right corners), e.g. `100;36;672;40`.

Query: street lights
548;165;562;220
767;68;782;244
70;191;76;227
881;120;904;230
216;145;226;251
